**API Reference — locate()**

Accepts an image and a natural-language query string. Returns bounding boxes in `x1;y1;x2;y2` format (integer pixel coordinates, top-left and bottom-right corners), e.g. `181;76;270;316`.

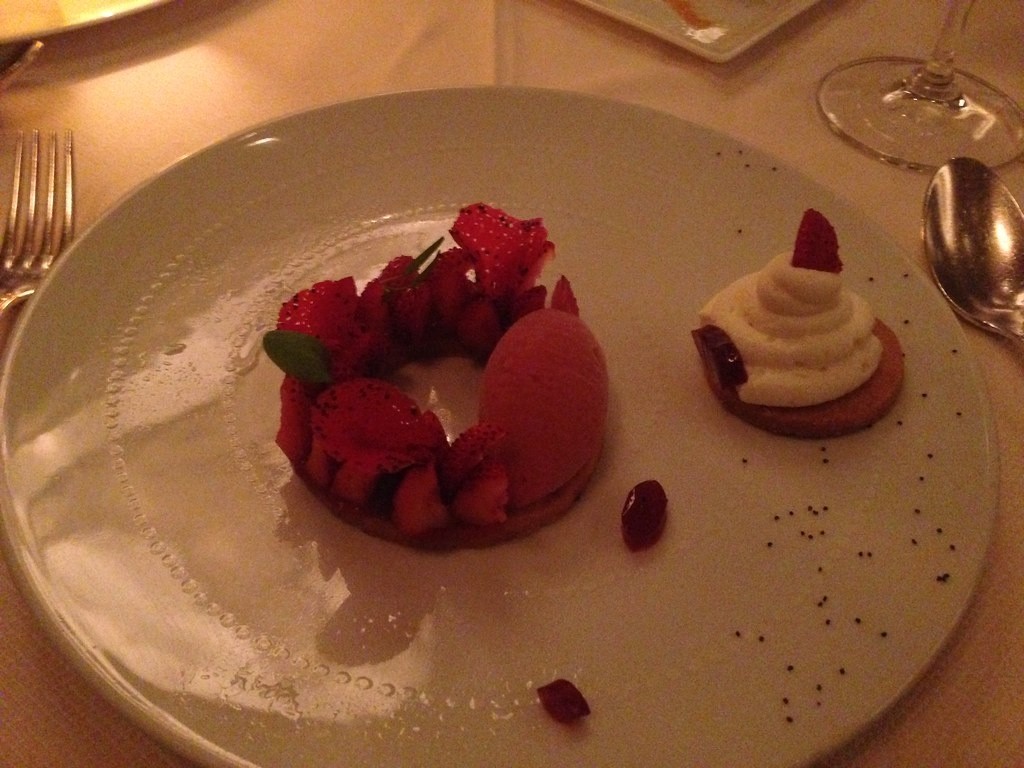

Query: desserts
261;201;607;550
692;208;906;441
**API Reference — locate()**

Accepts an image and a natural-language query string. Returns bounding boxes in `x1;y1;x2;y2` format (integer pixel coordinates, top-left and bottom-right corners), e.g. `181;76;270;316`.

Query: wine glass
816;0;1024;172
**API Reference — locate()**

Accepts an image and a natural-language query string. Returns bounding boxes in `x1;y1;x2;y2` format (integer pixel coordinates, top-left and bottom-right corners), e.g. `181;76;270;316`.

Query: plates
0;0;172;44
0;85;997;768
578;0;816;63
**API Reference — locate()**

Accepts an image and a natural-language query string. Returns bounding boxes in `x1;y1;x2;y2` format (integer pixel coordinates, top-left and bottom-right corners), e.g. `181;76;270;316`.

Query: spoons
920;158;1024;349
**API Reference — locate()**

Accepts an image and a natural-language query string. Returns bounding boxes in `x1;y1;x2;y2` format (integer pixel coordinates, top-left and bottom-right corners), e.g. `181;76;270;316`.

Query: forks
0;128;73;314
0;41;45;90
